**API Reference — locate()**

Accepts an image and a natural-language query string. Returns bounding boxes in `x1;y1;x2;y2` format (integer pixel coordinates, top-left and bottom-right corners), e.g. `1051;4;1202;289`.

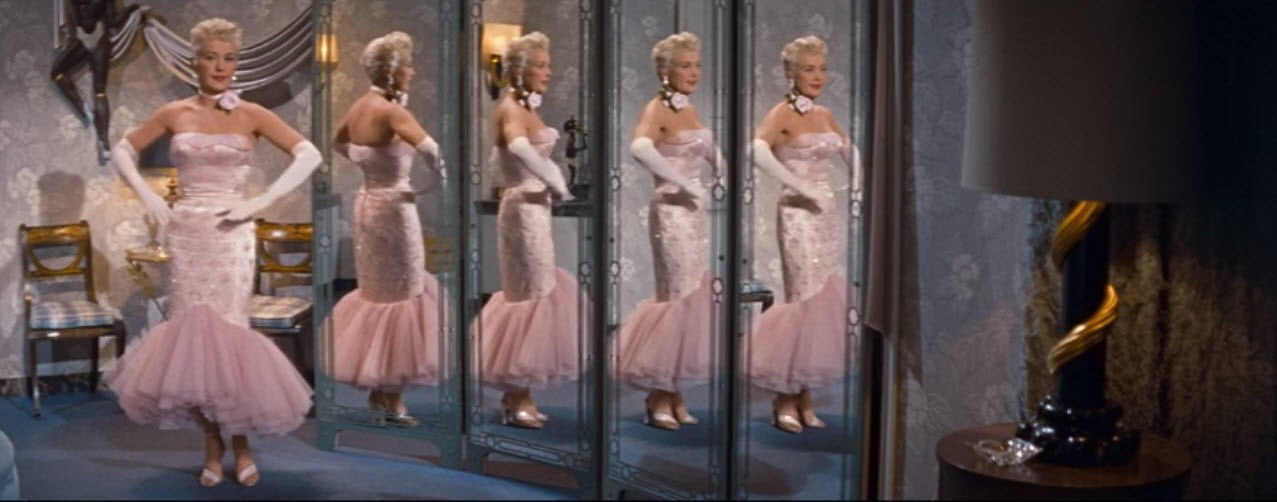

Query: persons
750;34;864;433
469;28;596;429
319;29;459;425
110;17;324;487
607;29;723;431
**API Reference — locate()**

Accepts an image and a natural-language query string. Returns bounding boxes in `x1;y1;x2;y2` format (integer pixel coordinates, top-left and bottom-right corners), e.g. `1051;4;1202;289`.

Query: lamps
960;0;1227;470
315;14;338;85
139;132;180;208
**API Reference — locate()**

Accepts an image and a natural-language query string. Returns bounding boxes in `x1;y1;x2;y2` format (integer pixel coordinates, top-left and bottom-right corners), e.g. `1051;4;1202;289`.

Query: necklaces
782;87;817;114
368;84;410;108
196;87;241;111
505;84;543;110
657;86;689;110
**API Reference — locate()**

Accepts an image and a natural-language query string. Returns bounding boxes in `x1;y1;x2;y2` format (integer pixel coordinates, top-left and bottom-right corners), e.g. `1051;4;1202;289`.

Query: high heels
644;398;679;429
233;441;259;486
792;395;826;428
200;432;227;487
367;395;417;426
500;392;543;428
531;406;547;421
771;398;803;433
675;410;698;424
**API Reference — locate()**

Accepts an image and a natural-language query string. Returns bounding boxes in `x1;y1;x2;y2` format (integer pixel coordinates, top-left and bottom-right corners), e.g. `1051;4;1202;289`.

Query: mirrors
315;1;890;502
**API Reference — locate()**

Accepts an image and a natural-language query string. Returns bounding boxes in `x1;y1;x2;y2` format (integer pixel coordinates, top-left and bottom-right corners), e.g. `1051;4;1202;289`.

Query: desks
936;423;1197;502
127;248;172;324
476;200;593;217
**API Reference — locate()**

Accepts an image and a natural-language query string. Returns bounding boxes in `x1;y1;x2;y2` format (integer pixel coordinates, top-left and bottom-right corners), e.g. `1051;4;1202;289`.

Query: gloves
747;138;821;204
630;137;706;197
416;137;447;187
111;137;175;226
214;139;323;221
508;137;568;197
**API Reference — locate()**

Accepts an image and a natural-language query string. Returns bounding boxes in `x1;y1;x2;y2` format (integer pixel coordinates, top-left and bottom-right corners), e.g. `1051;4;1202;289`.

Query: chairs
18;220;126;418
245;218;315;379
